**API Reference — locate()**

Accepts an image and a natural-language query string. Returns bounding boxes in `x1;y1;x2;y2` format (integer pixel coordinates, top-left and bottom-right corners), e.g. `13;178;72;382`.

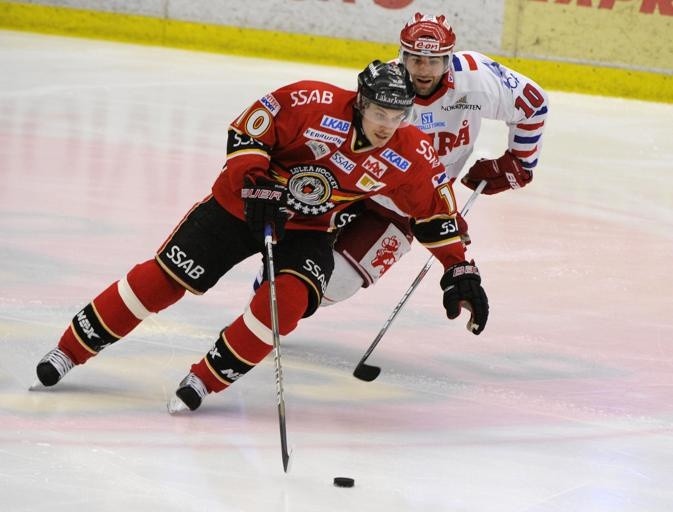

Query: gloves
460;150;533;196
440;258;490;336
242;173;289;232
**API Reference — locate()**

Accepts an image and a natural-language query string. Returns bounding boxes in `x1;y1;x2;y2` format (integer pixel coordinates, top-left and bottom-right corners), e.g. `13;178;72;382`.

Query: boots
176;371;207;412
36;347;75;388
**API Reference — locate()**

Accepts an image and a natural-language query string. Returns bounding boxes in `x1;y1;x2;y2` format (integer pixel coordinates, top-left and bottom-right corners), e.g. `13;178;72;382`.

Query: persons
29;60;489;414
253;12;549;305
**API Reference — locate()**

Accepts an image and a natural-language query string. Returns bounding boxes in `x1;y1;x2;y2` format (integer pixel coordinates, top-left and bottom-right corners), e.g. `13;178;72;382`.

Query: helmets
399;12;456;56
353;59;416;108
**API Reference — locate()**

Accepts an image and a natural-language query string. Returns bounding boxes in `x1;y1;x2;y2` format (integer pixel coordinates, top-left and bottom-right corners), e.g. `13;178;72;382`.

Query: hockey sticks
264;220;295;473
353;180;488;382
